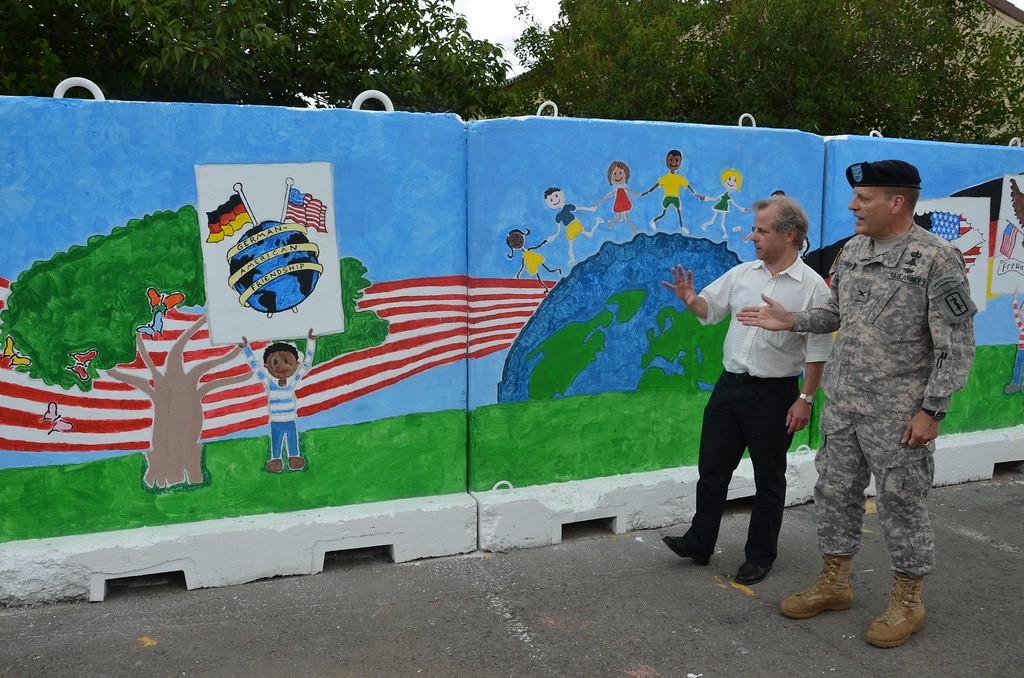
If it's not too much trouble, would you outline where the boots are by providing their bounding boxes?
[781,553,852,618]
[865,572,925,648]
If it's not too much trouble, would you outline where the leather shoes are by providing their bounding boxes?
[662,536,711,565]
[734,559,773,584]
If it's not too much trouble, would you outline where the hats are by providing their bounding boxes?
[846,160,922,189]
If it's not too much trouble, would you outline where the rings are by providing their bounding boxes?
[922,441,930,446]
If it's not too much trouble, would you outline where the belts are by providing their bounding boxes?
[723,369,798,385]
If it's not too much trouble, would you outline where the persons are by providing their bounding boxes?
[662,159,977,648]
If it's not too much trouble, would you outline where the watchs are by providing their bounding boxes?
[800,392,814,404]
[922,409,947,421]
